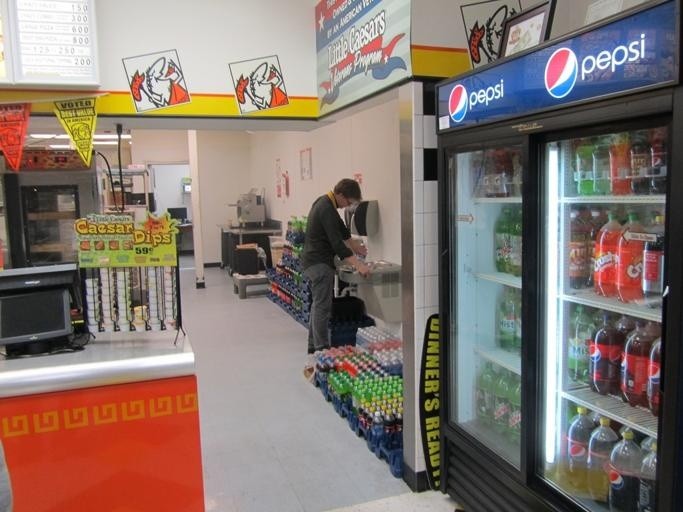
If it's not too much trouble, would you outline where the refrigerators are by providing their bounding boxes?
[432,0,681,508]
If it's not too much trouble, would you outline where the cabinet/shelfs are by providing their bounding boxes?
[220,217,282,275]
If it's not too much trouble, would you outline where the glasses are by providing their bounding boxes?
[347,199,352,206]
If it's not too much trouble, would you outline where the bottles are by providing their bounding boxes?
[609,131,631,195]
[615,315,634,334]
[567,406,597,495]
[286,215,308,249]
[576,137,597,195]
[619,320,657,410]
[472,147,524,198]
[588,317,603,393]
[647,333,663,418]
[587,417,622,504]
[640,435,657,453]
[584,306,595,318]
[593,313,626,395]
[567,305,597,384]
[509,207,524,278]
[629,130,651,195]
[651,127,669,194]
[305,326,406,480]
[591,411,602,427]
[593,134,612,195]
[598,209,613,228]
[514,290,522,350]
[642,216,666,309]
[283,244,304,275]
[508,375,521,446]
[594,213,623,298]
[645,211,660,226]
[615,213,644,304]
[476,362,497,427]
[638,443,658,512]
[608,431,641,512]
[266,265,311,323]
[492,206,517,274]
[569,207,600,289]
[498,286,517,352]
[626,320,643,345]
[491,369,514,435]
[571,138,579,196]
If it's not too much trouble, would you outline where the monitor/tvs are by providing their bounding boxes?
[167,207,187,219]
[1,262,87,332]
[0,288,73,354]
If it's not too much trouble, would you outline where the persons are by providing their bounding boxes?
[302,179,370,354]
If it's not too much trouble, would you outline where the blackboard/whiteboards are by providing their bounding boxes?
[146,162,195,253]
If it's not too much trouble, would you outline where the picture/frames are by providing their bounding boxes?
[496,0,557,58]
